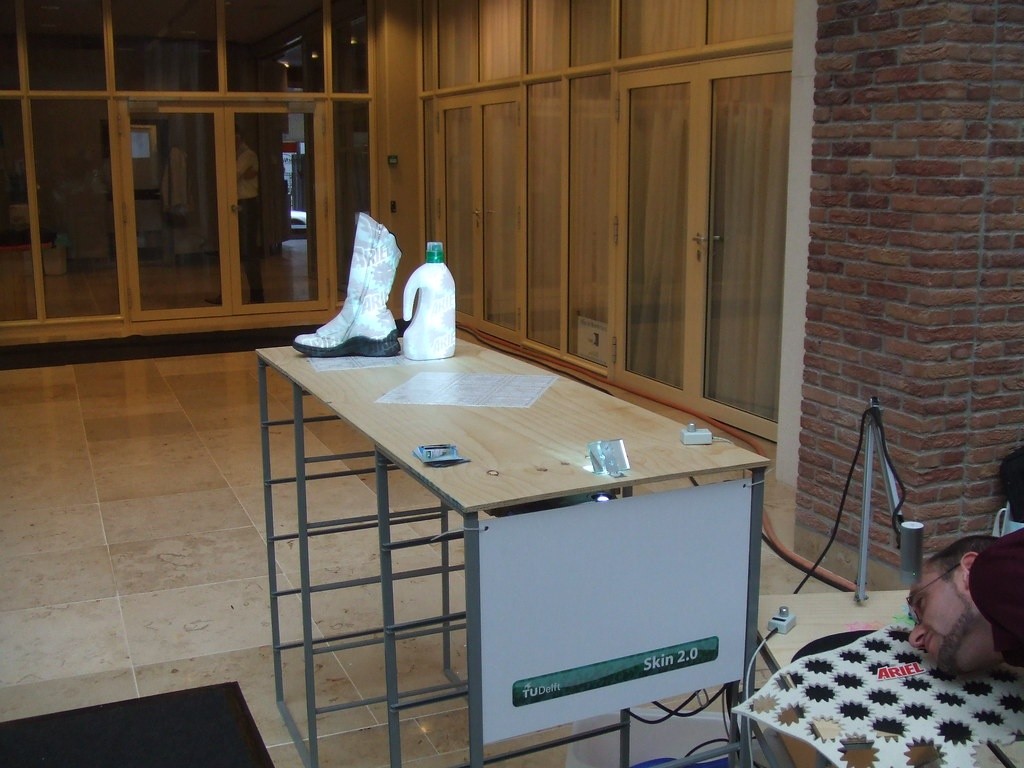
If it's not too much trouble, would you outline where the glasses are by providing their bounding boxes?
[906,563,961,626]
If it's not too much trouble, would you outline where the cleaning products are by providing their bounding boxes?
[401,239,458,362]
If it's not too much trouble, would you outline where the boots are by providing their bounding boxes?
[292,212,402,358]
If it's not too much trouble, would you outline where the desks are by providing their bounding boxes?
[262,337,771,768]
[757,590,1024,768]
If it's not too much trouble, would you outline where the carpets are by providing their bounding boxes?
[0,681,274,768]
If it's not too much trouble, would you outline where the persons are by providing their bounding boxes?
[907,527,1024,678]
[204,122,266,306]
